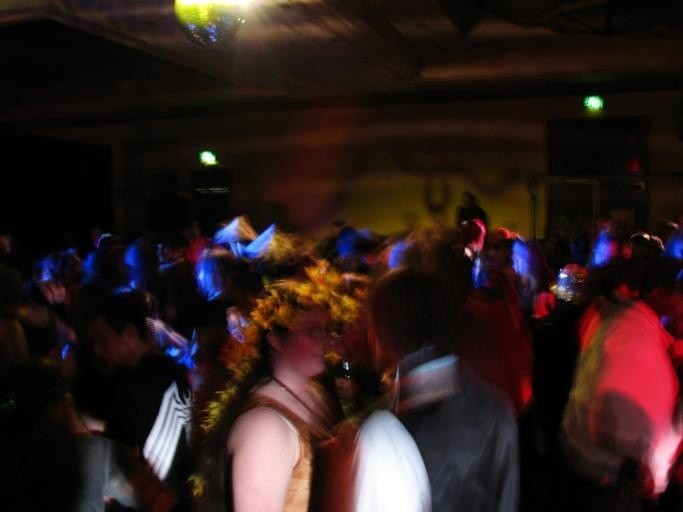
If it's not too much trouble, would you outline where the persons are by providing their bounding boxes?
[0,154,683,512]
